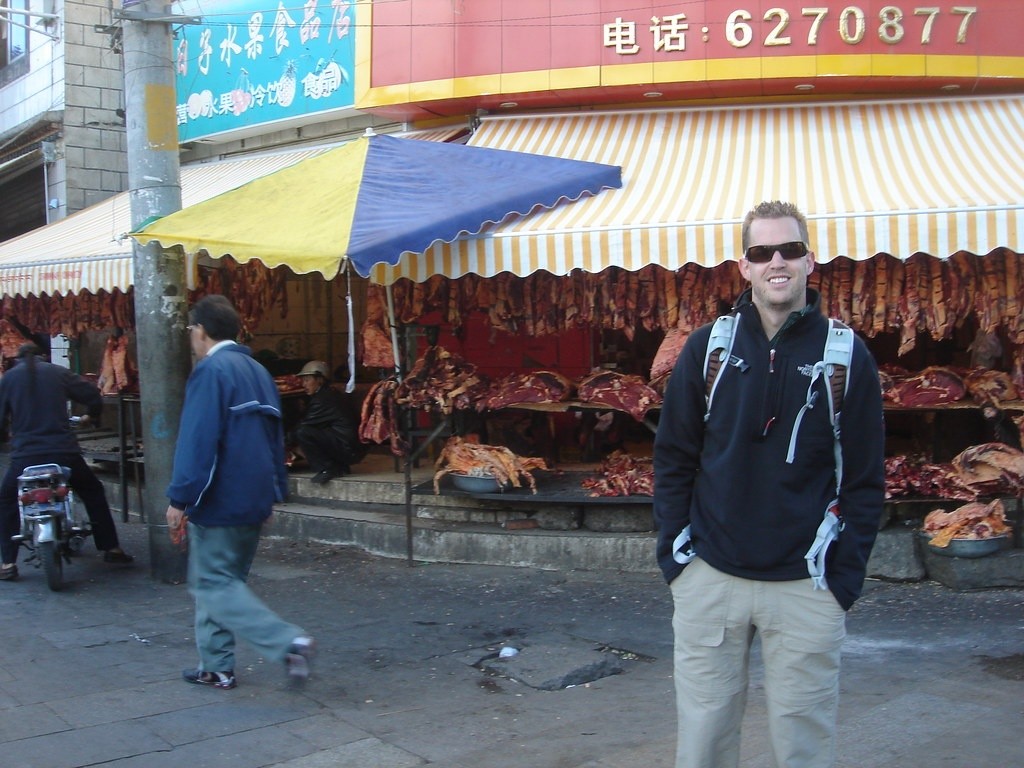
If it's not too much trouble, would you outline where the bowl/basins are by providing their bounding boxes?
[928,534,1009,559]
[450,472,518,493]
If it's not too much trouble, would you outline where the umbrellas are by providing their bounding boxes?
[109,126,623,382]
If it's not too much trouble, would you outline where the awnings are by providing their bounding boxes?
[0,124,465,300]
[369,96,1024,285]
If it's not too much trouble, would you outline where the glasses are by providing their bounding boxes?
[185,323,200,336]
[742,241,809,264]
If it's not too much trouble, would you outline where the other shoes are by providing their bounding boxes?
[102,550,133,566]
[0,564,19,581]
[311,468,339,482]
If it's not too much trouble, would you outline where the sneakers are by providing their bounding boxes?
[181,667,235,689]
[276,636,318,693]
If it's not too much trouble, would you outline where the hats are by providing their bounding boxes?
[296,361,332,382]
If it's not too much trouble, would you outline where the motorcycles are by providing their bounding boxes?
[11,415,88,592]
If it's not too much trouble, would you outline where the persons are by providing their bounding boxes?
[653,203,881,768]
[285,360,366,484]
[166,295,314,689]
[0,342,133,580]
